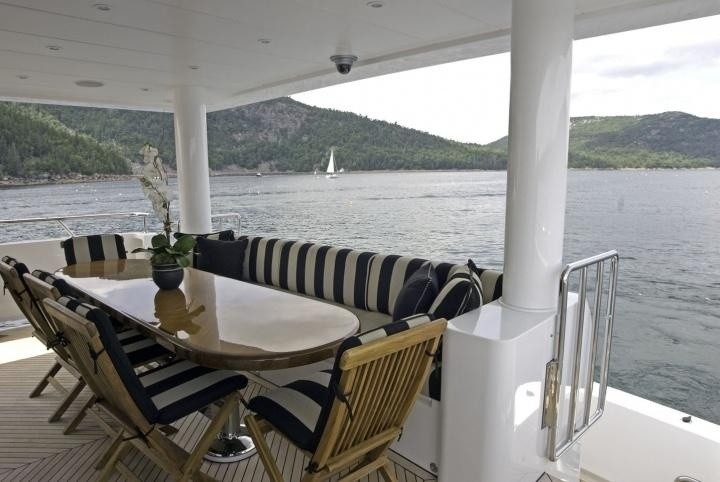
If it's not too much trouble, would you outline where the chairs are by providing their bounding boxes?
[244,313,447,482]
[23,268,176,423]
[59,234,127,264]
[1,257,44,343]
[42,294,249,481]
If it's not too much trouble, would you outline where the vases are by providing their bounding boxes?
[150,262,184,290]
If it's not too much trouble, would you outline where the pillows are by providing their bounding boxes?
[393,264,437,321]
[193,234,248,281]
[174,230,234,251]
[427,259,483,363]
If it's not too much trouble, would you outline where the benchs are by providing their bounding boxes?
[188,234,504,478]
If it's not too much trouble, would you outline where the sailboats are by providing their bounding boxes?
[325,149,340,179]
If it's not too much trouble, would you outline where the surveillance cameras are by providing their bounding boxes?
[330,55,358,75]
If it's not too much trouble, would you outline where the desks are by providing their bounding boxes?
[55,258,361,463]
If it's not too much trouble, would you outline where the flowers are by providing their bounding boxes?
[125,141,203,268]
